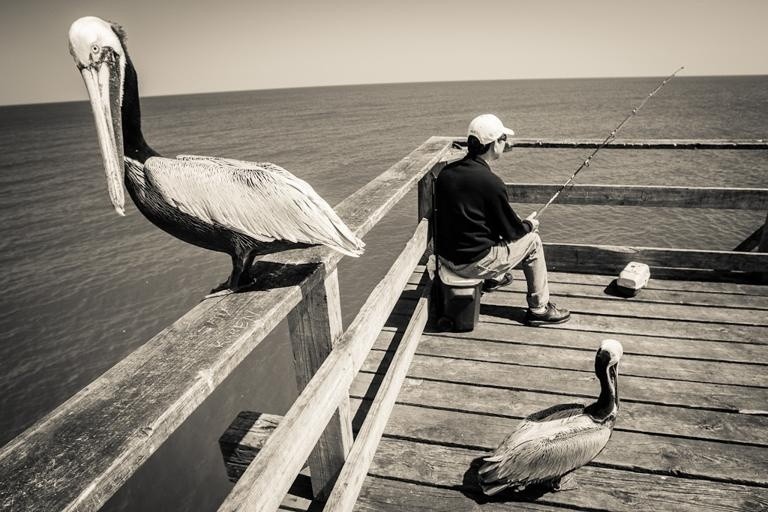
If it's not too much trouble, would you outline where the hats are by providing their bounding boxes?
[466,113,515,145]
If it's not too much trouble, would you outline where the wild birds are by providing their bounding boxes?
[67,14,368,303]
[473,336,624,498]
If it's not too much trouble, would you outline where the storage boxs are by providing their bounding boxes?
[614,260,653,297]
[438,260,488,331]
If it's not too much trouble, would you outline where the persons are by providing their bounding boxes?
[430,113,571,327]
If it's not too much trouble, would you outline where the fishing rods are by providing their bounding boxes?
[535,65,683,220]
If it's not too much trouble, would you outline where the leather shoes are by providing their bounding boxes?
[525,302,571,327]
[483,273,513,292]
